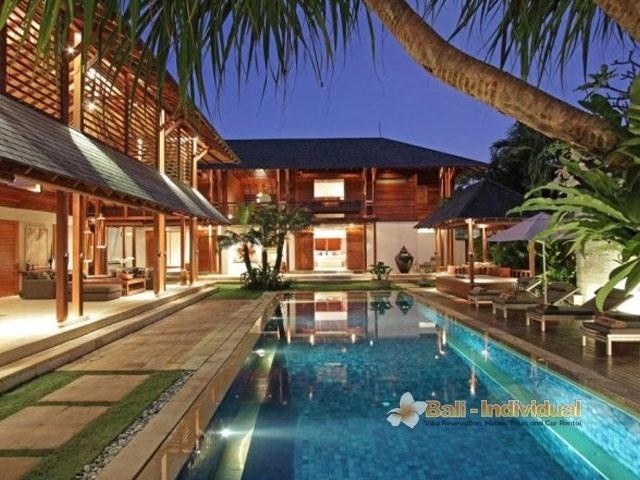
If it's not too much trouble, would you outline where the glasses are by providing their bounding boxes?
[96,200,106,220]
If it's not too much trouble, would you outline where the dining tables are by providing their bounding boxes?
[368,261,393,285]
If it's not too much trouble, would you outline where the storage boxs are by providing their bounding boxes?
[66,283,122,301]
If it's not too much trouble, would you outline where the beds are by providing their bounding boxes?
[487,210,571,305]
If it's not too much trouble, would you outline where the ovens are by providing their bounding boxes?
[435,272,519,300]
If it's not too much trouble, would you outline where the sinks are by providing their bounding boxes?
[21,269,72,299]
[117,267,153,290]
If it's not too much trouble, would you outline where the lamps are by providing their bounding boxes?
[579,315,640,355]
[492,282,580,319]
[467,278,543,309]
[525,286,634,332]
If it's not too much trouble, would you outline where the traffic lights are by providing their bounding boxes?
[447,261,512,277]
[34,271,57,280]
[123,267,152,280]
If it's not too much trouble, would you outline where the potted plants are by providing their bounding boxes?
[24,225,49,265]
